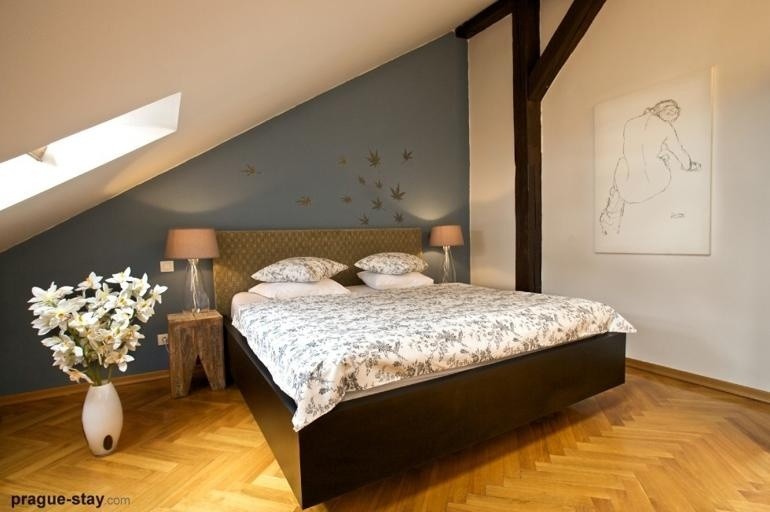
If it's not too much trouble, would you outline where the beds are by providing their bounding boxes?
[210,223,638,511]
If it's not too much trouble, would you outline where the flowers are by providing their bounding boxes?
[28,262,171,383]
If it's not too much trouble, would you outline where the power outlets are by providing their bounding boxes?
[156,332,169,346]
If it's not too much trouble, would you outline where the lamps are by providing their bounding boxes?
[163,226,222,313]
[427,225,465,283]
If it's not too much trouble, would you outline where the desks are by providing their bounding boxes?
[165,308,228,398]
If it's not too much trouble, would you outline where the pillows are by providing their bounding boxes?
[353,251,431,276]
[248,278,352,300]
[354,270,437,291]
[248,255,351,283]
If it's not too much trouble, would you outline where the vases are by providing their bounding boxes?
[80,385,126,457]
